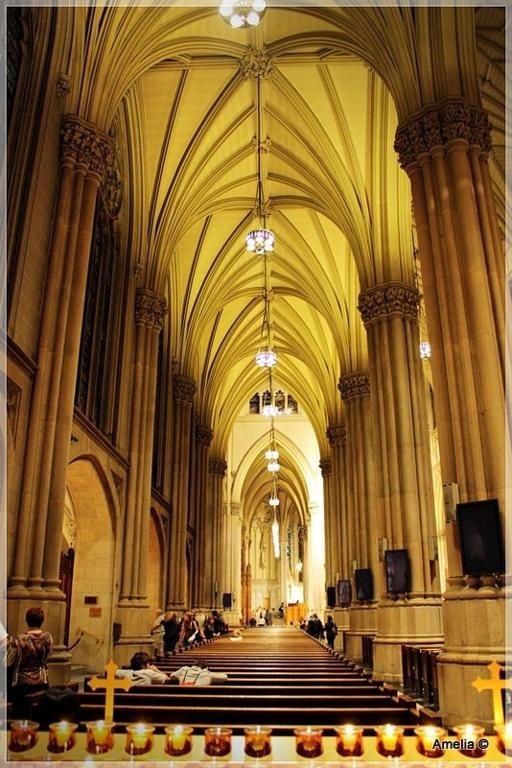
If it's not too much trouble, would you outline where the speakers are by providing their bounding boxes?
[327,587,335,606]
[385,548,410,593]
[223,593,232,607]
[355,568,373,600]
[338,580,351,604]
[456,498,505,576]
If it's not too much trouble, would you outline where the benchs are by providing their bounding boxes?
[5,621,445,734]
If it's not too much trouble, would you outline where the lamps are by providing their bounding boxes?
[217,0,281,419]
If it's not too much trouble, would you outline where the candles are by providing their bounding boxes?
[8,718,512,755]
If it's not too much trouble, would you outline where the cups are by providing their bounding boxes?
[11,717,511,759]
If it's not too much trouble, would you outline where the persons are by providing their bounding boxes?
[115,652,166,685]
[170,659,228,687]
[150,600,337,654]
[1,608,54,720]
